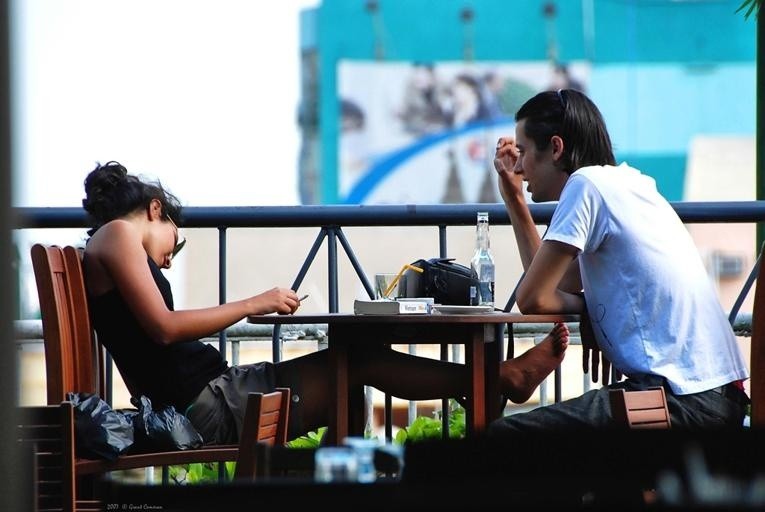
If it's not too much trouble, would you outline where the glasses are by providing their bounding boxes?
[167,214,186,259]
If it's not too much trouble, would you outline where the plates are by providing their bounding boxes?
[432,305,494,314]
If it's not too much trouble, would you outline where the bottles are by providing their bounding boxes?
[470,211,494,312]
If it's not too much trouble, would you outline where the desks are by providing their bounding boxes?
[248,306,583,448]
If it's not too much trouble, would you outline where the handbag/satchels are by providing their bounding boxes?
[398,258,482,306]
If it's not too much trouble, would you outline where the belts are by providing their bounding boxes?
[713,384,751,407]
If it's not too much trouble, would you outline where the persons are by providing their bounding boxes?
[395,62,486,135]
[340,100,364,134]
[548,66,584,93]
[83,161,570,449]
[490,89,749,483]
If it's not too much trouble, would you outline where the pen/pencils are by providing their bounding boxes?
[298,295,309,302]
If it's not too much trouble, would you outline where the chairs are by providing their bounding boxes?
[14,245,292,512]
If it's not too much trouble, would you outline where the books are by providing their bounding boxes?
[354,299,427,316]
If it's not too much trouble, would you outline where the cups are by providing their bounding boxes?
[375,274,408,301]
